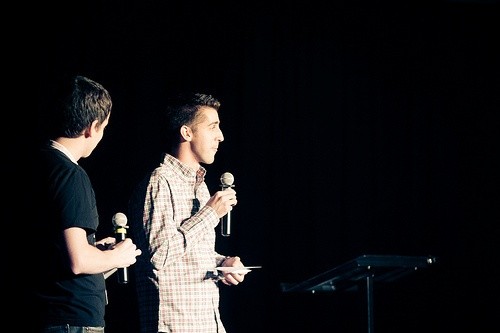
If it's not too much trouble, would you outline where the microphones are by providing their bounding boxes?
[220,172,234,237]
[112,213,129,283]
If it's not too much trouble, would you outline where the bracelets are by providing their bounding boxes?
[218,255,231,287]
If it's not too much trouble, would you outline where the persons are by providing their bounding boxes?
[0,78,142,333]
[142,93,249,333]
